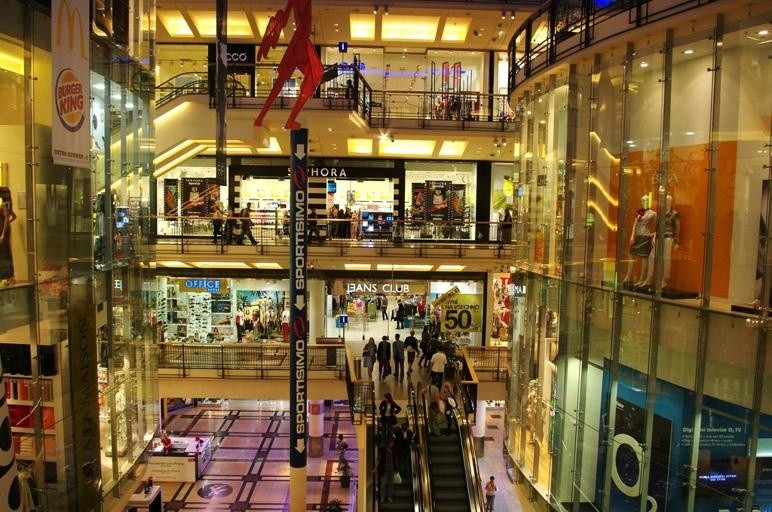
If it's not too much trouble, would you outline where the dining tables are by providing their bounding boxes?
[335,458,359,488]
[319,498,348,512]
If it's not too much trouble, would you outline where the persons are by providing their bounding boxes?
[236,309,244,343]
[395,300,404,329]
[434,97,472,120]
[161,435,173,455]
[362,325,457,505]
[0,185,17,286]
[211,202,259,246]
[283,205,351,243]
[622,195,657,287]
[214,327,220,339]
[98,204,116,264]
[499,208,513,244]
[345,79,353,99]
[484,476,498,512]
[336,434,350,470]
[380,295,389,320]
[638,194,680,289]
[281,308,290,342]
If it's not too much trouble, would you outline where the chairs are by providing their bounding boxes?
[380,496,386,504]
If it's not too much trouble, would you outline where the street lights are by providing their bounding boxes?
[213,214,222,226]
[364,356,373,367]
[384,361,391,376]
[393,471,402,485]
[430,402,437,410]
[434,412,446,424]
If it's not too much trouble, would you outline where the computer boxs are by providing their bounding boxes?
[391,136,394,142]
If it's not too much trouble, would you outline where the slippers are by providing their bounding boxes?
[239,209,250,225]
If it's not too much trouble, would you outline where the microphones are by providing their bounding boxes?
[403,239,476,258]
[157,234,223,254]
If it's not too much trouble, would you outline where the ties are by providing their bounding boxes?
[360,210,394,237]
[328,183,336,193]
[212,301,231,312]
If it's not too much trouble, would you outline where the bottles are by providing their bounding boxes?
[384,436,394,446]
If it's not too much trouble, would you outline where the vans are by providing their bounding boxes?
[4,277,13,286]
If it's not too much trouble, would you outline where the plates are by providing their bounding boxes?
[1,309,74,512]
[167,285,233,339]
[143,276,167,342]
